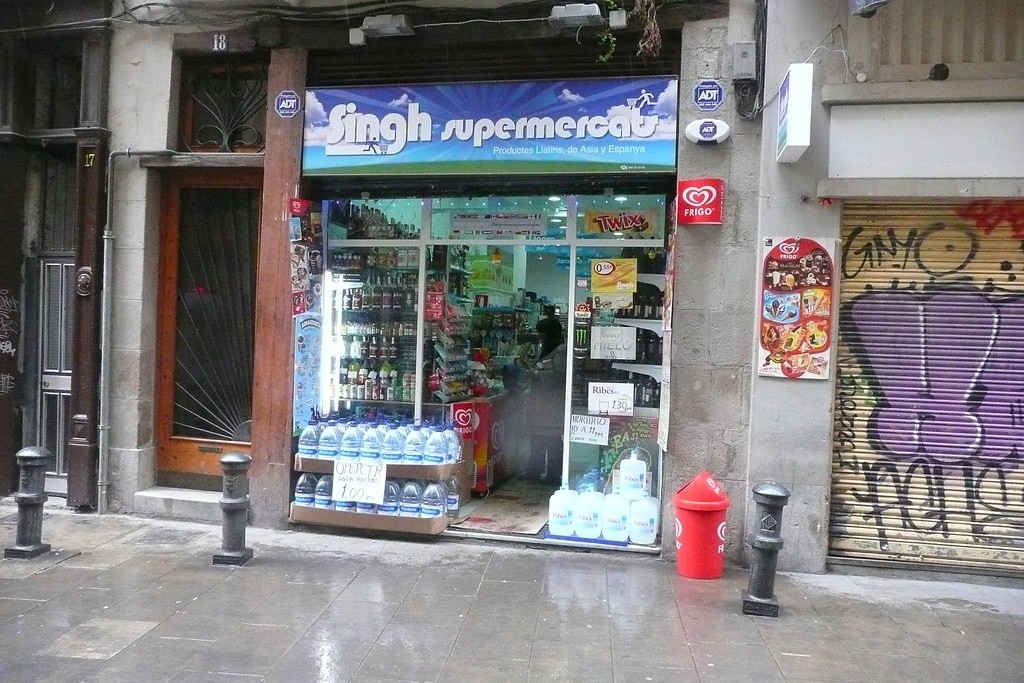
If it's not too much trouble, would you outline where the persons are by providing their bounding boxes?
[517,317,577,484]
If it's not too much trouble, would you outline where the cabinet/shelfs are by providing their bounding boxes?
[327,216,532,502]
[609,272,665,418]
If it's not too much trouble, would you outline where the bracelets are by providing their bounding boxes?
[534,370,538,376]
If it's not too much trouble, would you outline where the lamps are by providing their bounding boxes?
[546,3,603,28]
[360,16,415,38]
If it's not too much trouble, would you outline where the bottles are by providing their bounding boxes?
[615,287,664,319]
[294,473,463,519]
[622,236,666,274]
[331,313,417,402]
[328,246,419,311]
[347,204,419,239]
[548,450,658,544]
[298,414,461,464]
[636,329,663,364]
[625,371,661,407]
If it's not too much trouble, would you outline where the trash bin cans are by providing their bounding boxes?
[674,472,730,580]
